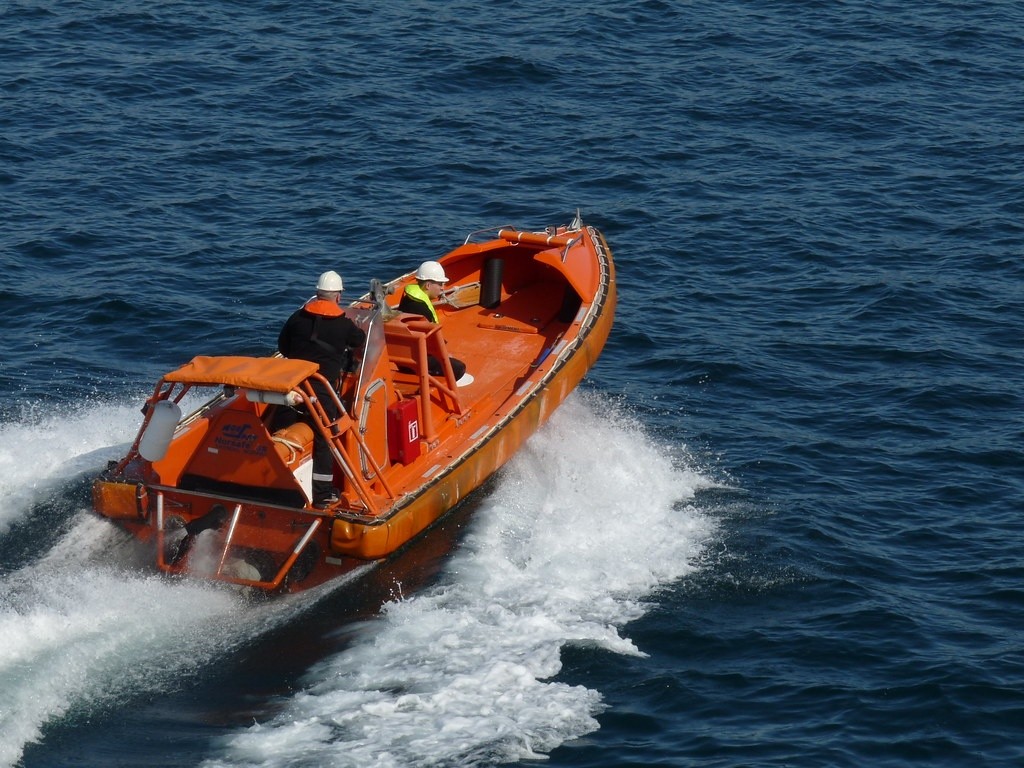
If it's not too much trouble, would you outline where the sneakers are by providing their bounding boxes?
[314,488,340,509]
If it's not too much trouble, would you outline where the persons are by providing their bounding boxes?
[398,260,466,382]
[278,271,367,505]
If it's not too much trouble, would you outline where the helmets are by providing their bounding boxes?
[316,271,346,292]
[414,261,449,283]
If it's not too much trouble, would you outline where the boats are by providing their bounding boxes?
[87,207,619,593]
